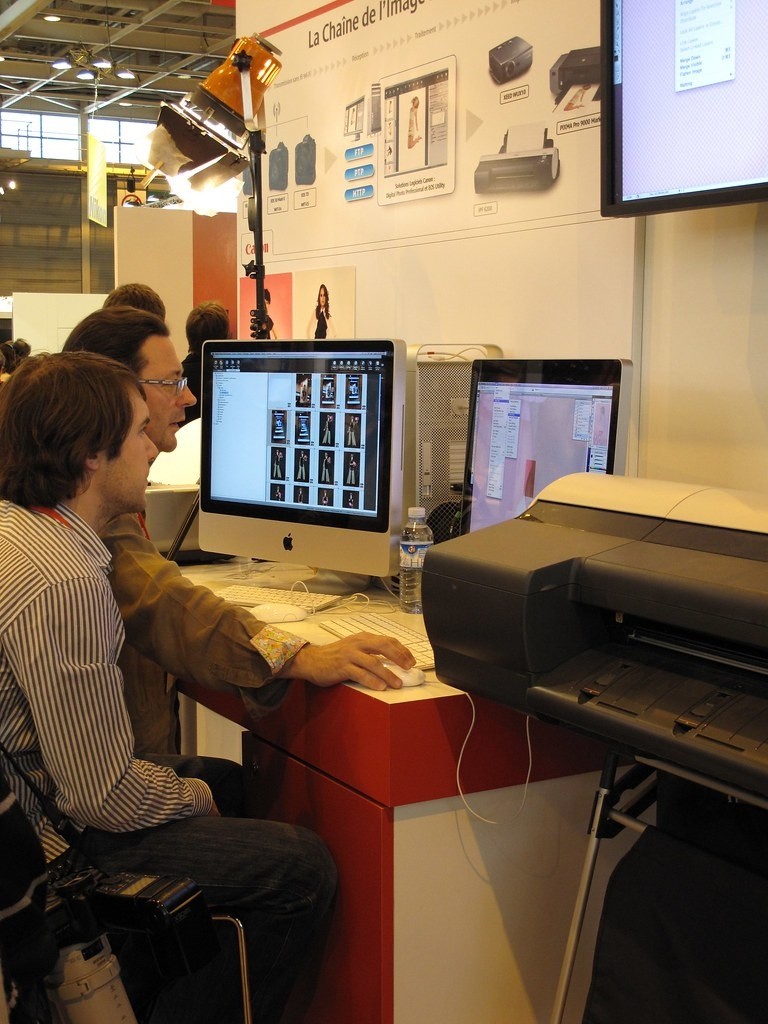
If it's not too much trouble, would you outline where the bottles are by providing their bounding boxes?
[399,505,434,615]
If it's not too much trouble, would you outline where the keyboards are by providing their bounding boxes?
[214,584,343,614]
[317,612,436,671]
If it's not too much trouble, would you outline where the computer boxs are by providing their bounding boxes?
[368,343,504,590]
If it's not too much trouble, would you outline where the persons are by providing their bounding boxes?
[1,285,416,1023]
[180,301,228,427]
[306,284,336,339]
[408,97,422,150]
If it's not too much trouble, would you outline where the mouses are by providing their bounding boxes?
[250,603,308,623]
[368,662,426,687]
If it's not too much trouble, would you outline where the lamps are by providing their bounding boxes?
[145,31,283,341]
[126,166,136,193]
[52,59,136,82]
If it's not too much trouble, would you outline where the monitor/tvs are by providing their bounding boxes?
[200,339,409,598]
[459,357,633,537]
[600,0,768,218]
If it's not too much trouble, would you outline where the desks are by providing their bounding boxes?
[166,564,657,1024]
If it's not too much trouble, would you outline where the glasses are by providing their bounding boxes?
[135,377,188,396]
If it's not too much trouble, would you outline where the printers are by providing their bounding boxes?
[420,470,768,801]
[549,46,600,103]
[473,129,561,194]
